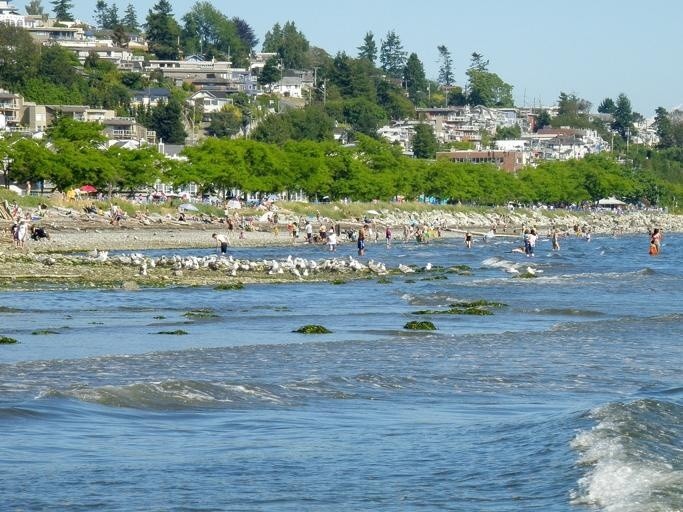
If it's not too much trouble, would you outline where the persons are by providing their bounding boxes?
[404,212,447,242]
[464,231,473,248]
[137,193,167,204]
[267,212,299,243]
[176,213,223,225]
[25,180,32,195]
[582,203,635,213]
[376,227,392,245]
[38,201,42,211]
[7,199,29,249]
[225,216,247,239]
[358,224,369,256]
[304,221,312,243]
[316,209,321,220]
[490,222,526,233]
[313,223,337,250]
[524,219,564,257]
[650,227,660,255]
[574,223,593,243]
[67,187,104,216]
[212,233,232,256]
